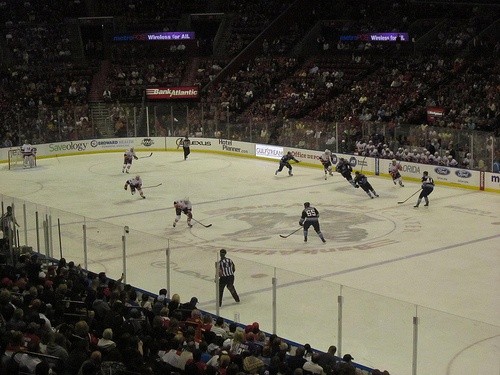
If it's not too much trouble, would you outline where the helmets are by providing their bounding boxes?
[304,202,310,207]
[383,144,450,158]
[325,149,330,153]
[392,159,396,164]
[184,195,189,201]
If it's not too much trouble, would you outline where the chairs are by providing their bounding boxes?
[22,299,89,375]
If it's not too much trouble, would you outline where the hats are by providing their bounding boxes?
[191,297,199,303]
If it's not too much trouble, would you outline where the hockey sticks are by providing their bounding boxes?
[398,188,422,204]
[279,226,303,238]
[141,183,162,189]
[182,211,212,228]
[134,152,153,160]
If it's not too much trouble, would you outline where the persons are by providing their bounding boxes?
[124,176,146,198]
[388,159,405,187]
[335,157,358,188]
[299,202,326,243]
[21,140,36,168]
[319,149,334,182]
[183,136,191,159]
[173,197,192,227]
[214,249,240,307]
[0,206,20,243]
[354,171,379,199]
[0,239,390,375]
[0,0,500,173]
[122,147,138,174]
[275,151,299,176]
[414,171,435,207]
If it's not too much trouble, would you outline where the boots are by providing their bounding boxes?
[321,237,326,243]
[304,236,307,241]
[372,191,379,197]
[368,193,374,199]
[424,201,429,206]
[413,202,419,207]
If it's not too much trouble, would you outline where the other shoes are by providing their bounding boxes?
[324,175,327,180]
[399,181,404,187]
[394,182,396,185]
[288,170,293,176]
[275,169,281,175]
[329,173,333,176]
[141,196,145,198]
[187,222,192,227]
[173,222,176,227]
